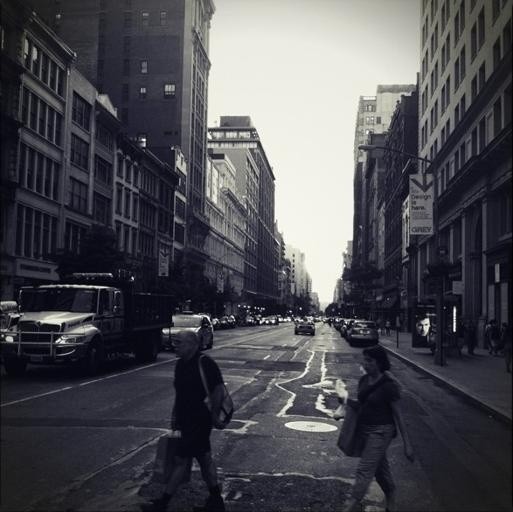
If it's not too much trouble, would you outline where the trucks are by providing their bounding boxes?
[0,274,174,377]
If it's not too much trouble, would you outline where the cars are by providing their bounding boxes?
[340,319,378,346]
[211,315,236,330]
[295,319,315,335]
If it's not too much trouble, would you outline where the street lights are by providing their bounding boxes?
[357,144,446,367]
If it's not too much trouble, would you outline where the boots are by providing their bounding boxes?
[192,484,228,512]
[140,492,171,512]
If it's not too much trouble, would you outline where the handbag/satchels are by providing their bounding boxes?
[152,434,191,482]
[204,384,233,430]
[337,403,369,458]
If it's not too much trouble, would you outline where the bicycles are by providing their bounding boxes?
[456,318,512,373]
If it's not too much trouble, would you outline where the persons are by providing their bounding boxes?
[375,314,386,336]
[498,322,513,372]
[139,328,227,512]
[414,313,432,347]
[463,318,478,354]
[487,320,498,357]
[384,315,392,337]
[332,342,419,512]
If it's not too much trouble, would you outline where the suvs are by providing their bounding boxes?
[161,314,214,352]
